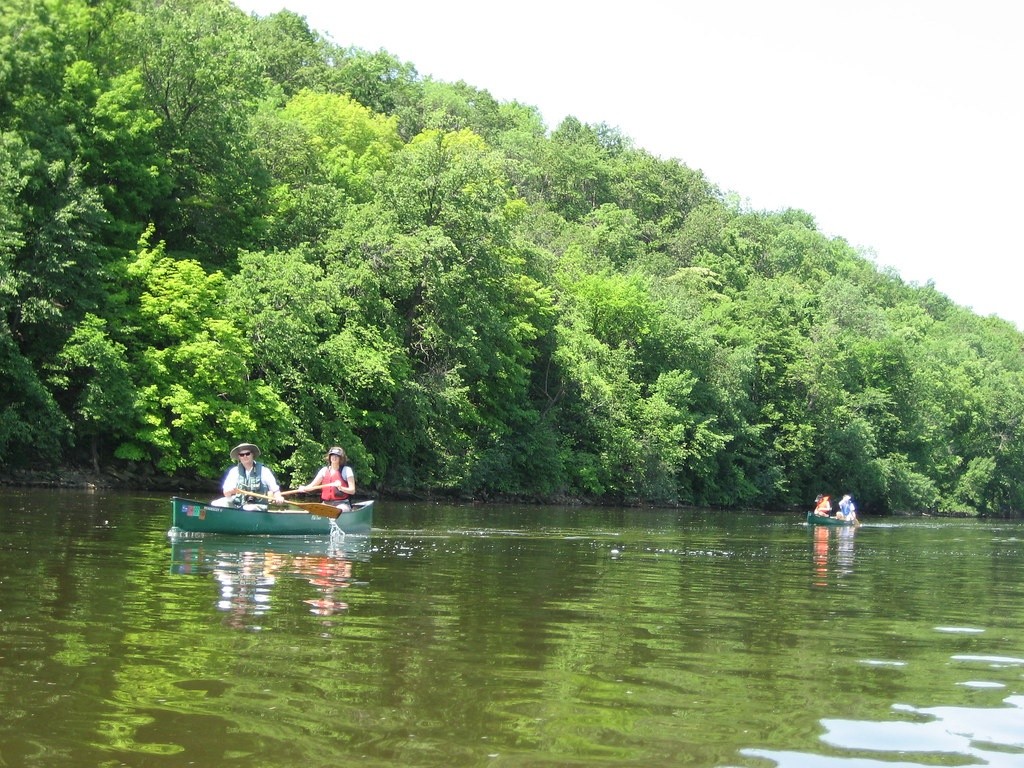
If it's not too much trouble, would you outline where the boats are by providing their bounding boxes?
[168,494,375,536]
[806,510,860,526]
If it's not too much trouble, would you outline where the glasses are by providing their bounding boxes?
[237,452,250,457]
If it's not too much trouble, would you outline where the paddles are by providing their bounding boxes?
[281,482,335,496]
[236,488,342,520]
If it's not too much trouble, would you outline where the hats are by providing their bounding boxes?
[230,442,261,462]
[329,447,344,456]
[844,496,851,500]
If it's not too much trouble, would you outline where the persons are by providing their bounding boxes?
[837,494,856,521]
[300,447,356,512]
[211,443,284,512]
[814,494,832,518]
